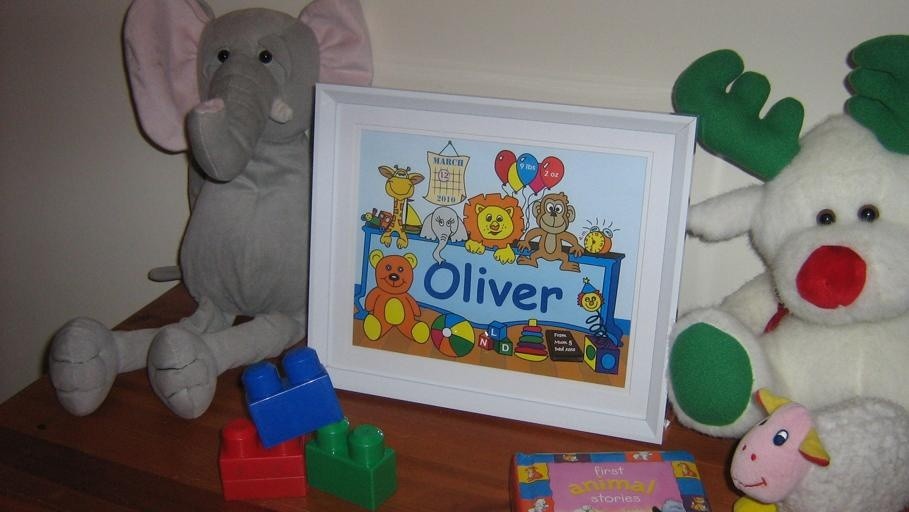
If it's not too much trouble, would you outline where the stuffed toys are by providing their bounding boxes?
[45,0,373,418]
[666,35,909,438]
[723,388,908,511]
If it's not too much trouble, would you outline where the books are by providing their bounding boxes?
[507,447,713,510]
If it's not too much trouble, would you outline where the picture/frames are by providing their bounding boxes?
[303,83,697,446]
